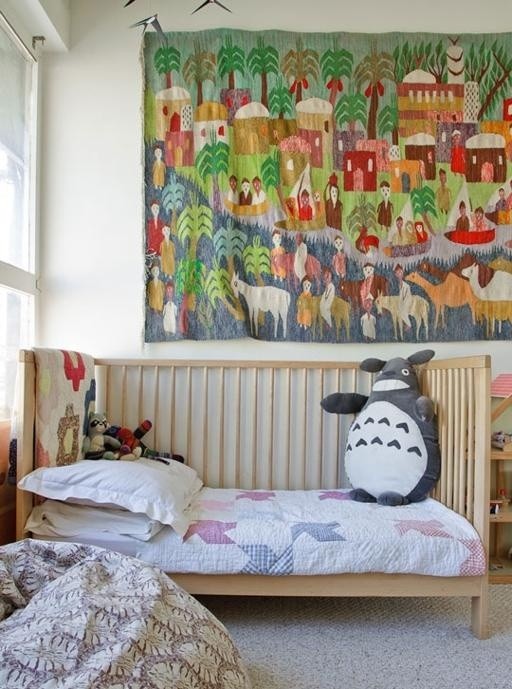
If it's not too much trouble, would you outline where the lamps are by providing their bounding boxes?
[490,374,512,400]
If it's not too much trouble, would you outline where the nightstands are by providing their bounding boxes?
[466,395,511,584]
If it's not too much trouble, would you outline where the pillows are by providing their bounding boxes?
[17,458,204,542]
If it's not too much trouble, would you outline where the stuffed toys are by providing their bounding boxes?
[106,419,184,461]
[320,347,442,506]
[82,412,121,461]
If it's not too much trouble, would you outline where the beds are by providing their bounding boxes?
[14,349,490,640]
